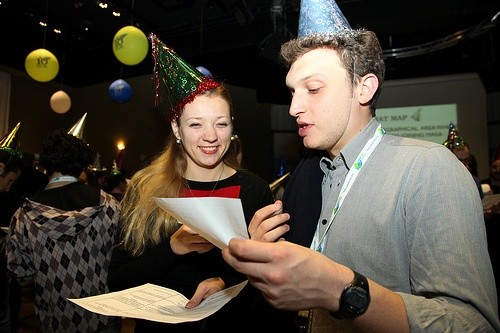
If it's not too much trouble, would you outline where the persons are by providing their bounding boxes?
[107,33,274,333]
[443,123,500,237]
[0,112,285,333]
[221,0,500,333]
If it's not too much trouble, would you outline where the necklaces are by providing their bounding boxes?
[183,163,225,198]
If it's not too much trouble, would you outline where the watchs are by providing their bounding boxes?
[328,269,371,324]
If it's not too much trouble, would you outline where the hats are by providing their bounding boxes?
[298,0,352,39]
[0,121,24,160]
[442,121,463,151]
[110,160,121,176]
[148,33,222,122]
[90,152,104,173]
[66,112,88,141]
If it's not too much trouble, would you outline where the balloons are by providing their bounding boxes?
[113,26,149,65]
[195,66,212,79]
[25,49,60,83]
[109,79,132,100]
[51,91,72,113]
